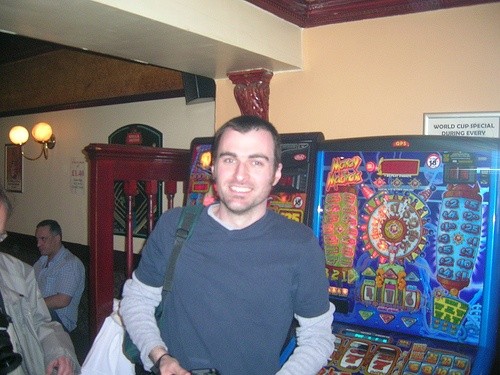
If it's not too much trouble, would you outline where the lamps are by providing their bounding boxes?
[9,122,56,160]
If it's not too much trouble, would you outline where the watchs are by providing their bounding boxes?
[150,353,174,375]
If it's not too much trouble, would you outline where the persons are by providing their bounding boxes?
[0,190,86,375]
[118,115,337,375]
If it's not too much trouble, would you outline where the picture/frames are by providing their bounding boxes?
[4,143,24,193]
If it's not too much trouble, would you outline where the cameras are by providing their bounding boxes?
[0,305,23,375]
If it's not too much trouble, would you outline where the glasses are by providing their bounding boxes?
[0,233,7,242]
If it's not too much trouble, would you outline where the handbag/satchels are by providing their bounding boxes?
[123,205,203,364]
